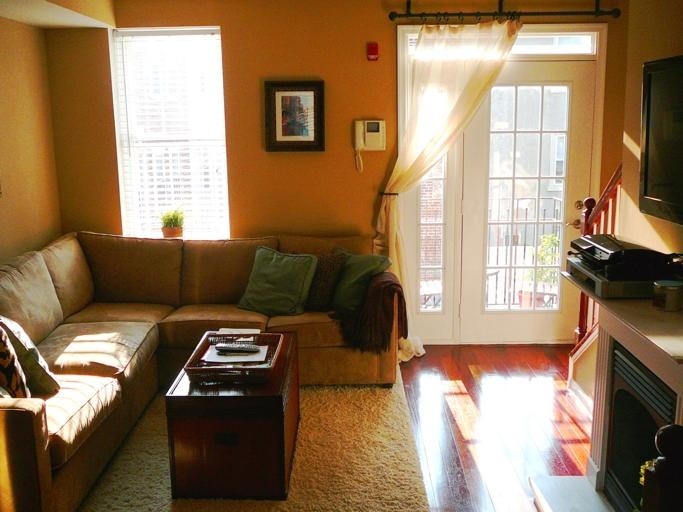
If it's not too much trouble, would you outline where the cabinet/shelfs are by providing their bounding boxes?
[559,271,683,512]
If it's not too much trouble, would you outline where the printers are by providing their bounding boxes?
[571,234,673,280]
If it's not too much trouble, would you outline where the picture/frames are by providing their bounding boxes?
[265,79,325,150]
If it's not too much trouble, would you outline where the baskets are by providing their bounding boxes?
[182,329,287,387]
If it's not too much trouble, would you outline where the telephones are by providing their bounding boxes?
[355,120,386,151]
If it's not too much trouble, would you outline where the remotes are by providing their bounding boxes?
[216,343,259,351]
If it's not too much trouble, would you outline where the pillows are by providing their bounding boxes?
[238,246,316,316]
[286,247,343,310]
[329,246,392,312]
[1,317,59,400]
[1,325,32,400]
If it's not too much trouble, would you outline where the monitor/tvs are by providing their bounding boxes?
[639,56,683,224]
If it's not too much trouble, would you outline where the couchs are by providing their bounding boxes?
[157,234,398,388]
[0,229,183,511]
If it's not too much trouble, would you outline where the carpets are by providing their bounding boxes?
[70,385,429,510]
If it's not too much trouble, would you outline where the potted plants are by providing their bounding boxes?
[162,212,185,238]
